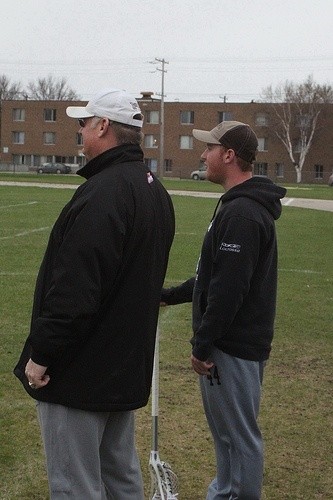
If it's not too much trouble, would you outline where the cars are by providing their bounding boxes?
[36,163,71,174]
[190,165,210,180]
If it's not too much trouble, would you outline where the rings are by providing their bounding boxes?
[28,382,36,389]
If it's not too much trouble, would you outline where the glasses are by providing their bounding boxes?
[77,118,111,127]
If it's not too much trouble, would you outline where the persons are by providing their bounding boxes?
[158,121,288,499]
[12,87,179,500]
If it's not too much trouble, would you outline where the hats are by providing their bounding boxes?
[65,89,144,128]
[192,121,258,163]
